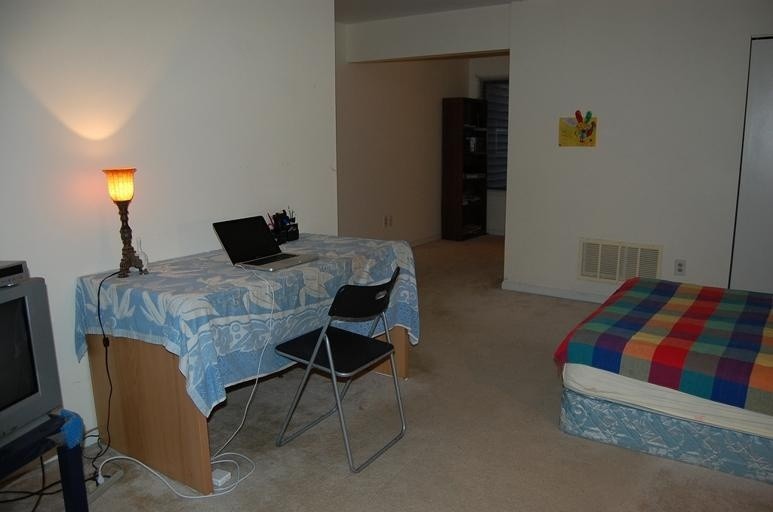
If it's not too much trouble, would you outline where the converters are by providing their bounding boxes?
[211,468,232,486]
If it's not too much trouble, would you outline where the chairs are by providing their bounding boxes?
[273,264,407,474]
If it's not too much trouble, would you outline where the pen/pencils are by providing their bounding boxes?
[269,207,297,231]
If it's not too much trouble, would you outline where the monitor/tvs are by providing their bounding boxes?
[0,276,63,451]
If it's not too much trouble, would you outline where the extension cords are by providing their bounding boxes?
[85,462,124,504]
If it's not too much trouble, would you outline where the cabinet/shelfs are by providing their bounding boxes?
[441,97,489,242]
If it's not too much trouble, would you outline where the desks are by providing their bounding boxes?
[1,415,94,510]
[79,232,415,497]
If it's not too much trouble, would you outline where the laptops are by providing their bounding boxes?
[212,214,320,273]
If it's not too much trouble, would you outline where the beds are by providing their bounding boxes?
[556,274,773,487]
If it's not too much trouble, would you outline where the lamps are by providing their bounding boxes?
[103,166,148,280]
